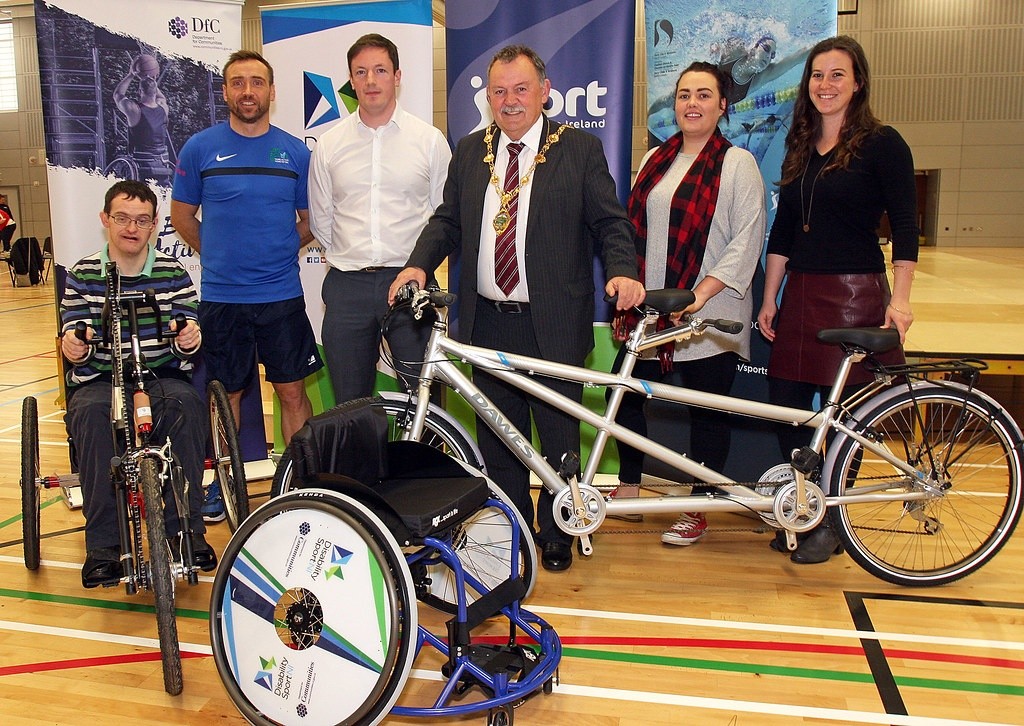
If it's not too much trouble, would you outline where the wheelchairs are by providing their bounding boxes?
[208,397,562,726]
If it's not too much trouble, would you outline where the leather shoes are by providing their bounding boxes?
[190,532,212,564]
[541,542,572,572]
[87,563,124,582]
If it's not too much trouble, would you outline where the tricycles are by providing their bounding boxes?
[19,260,249,695]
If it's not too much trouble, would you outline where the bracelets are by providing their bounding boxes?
[889,304,912,315]
[891,265,915,280]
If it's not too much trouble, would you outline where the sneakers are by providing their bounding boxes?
[602,490,644,521]
[660,509,708,545]
[200,476,238,522]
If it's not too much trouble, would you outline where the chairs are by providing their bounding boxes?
[41,236,53,282]
[0,236,45,288]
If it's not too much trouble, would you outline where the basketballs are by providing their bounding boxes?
[136,54,160,79]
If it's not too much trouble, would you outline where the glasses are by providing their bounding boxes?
[108,213,154,230]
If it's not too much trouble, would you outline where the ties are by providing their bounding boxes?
[494,142,526,298]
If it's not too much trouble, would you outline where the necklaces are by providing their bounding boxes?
[484,125,574,235]
[801,149,836,232]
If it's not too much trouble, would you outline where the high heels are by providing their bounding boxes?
[791,505,845,564]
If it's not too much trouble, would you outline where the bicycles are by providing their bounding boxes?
[271,279,1024,588]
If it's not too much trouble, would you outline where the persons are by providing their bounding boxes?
[0,196,16,252]
[305,33,455,412]
[57,182,218,585]
[757,39,919,564]
[114,65,170,167]
[388,45,646,571]
[602,64,767,544]
[171,51,326,524]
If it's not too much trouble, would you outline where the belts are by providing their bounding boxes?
[478,294,531,315]
